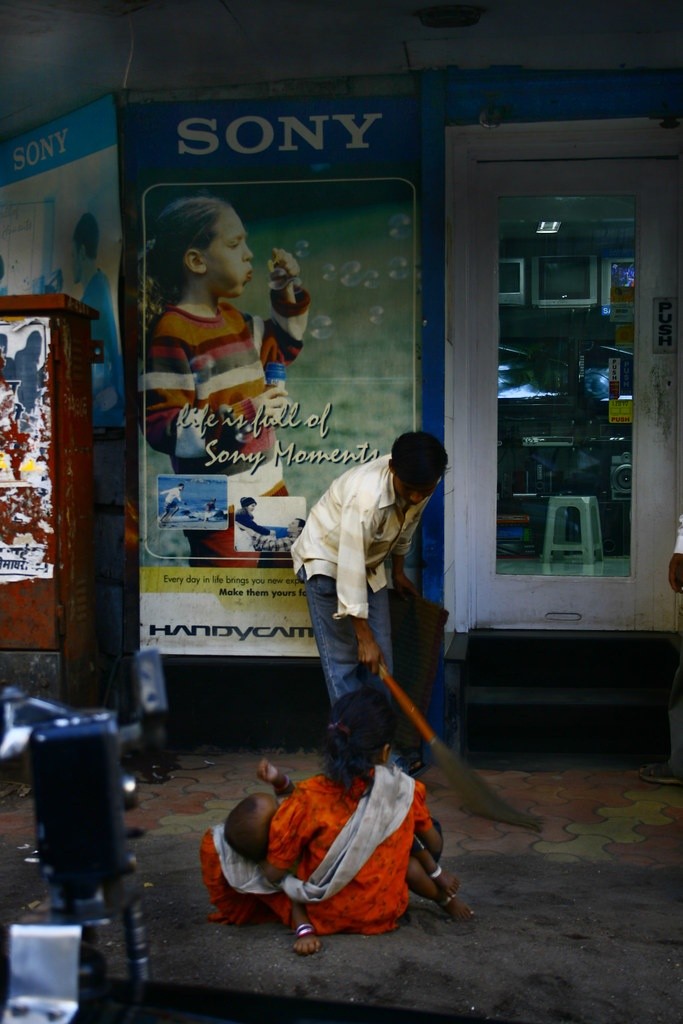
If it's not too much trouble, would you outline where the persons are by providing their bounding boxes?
[225,689,475,955]
[139,196,312,568]
[234,497,306,552]
[638,513,682,784]
[159,483,188,523]
[289,431,448,779]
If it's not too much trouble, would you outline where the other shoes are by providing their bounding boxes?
[638,763,683,785]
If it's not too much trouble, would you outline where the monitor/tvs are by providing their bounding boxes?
[497,337,609,416]
[531,256,598,307]
[498,258,525,305]
[601,257,635,307]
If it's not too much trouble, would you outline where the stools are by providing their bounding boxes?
[542,495,603,564]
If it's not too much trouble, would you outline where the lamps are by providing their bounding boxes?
[413,6,486,28]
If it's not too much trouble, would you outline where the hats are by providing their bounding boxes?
[240,497,257,507]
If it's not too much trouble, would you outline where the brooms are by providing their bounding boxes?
[377,659,550,833]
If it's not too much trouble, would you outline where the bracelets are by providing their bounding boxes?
[275,773,289,792]
[296,923,315,939]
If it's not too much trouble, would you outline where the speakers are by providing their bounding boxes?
[598,501,624,556]
[609,452,632,500]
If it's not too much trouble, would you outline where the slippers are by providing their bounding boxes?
[394,756,434,778]
